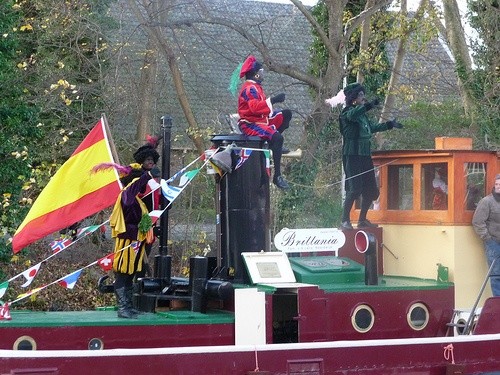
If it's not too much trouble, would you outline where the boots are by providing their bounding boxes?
[115,287,140,319]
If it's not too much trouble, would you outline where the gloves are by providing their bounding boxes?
[369,98,383,109]
[271,93,285,105]
[390,118,404,130]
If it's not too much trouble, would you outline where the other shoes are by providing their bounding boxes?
[357,219,378,228]
[342,220,354,230]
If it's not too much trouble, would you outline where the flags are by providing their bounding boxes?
[0,149,271,320]
[12,118,122,253]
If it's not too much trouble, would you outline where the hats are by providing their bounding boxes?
[240,55,257,78]
[133,143,160,164]
[120,169,144,187]
[343,83,364,104]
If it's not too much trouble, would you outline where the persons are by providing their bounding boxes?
[108,143,162,318]
[238,55,292,189]
[338,83,403,230]
[470,174,500,296]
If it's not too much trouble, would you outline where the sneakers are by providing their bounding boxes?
[273,174,290,190]
[282,147,289,155]
